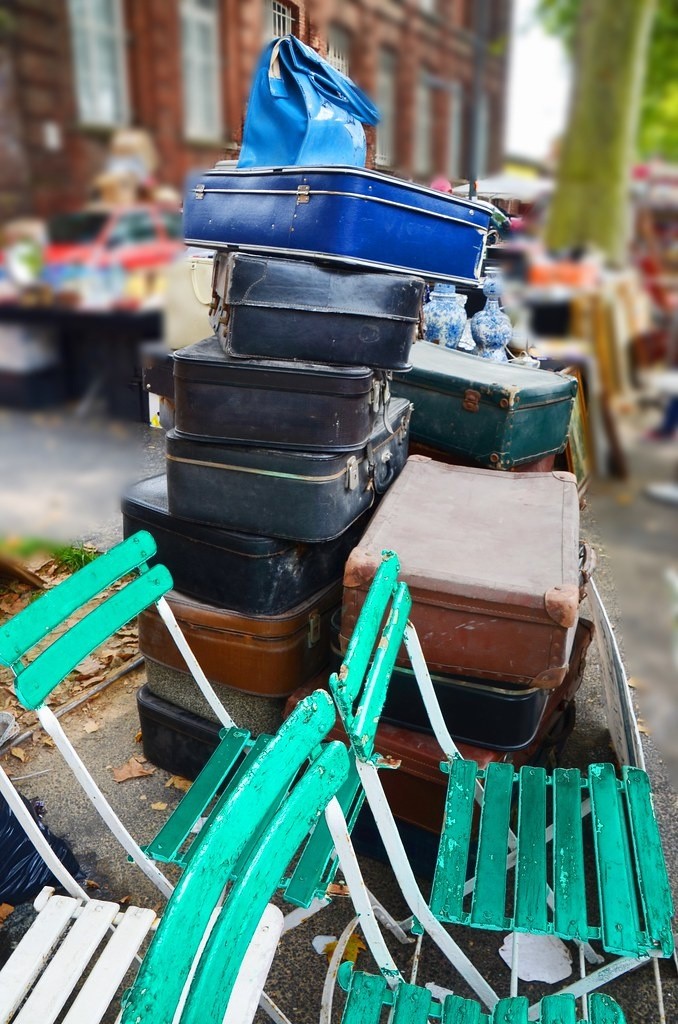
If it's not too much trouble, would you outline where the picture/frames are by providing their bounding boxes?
[556,365,596,499]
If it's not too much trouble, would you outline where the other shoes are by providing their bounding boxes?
[641,428,676,445]
[646,478,678,505]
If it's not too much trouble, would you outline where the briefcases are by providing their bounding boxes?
[117,164,509,800]
[388,338,580,472]
[275,454,596,898]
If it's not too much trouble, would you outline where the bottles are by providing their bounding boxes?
[470,266,513,364]
[420,281,469,350]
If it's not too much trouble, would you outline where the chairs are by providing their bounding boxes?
[2,530,677,1024]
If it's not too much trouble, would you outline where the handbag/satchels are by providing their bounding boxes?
[236,33,384,168]
